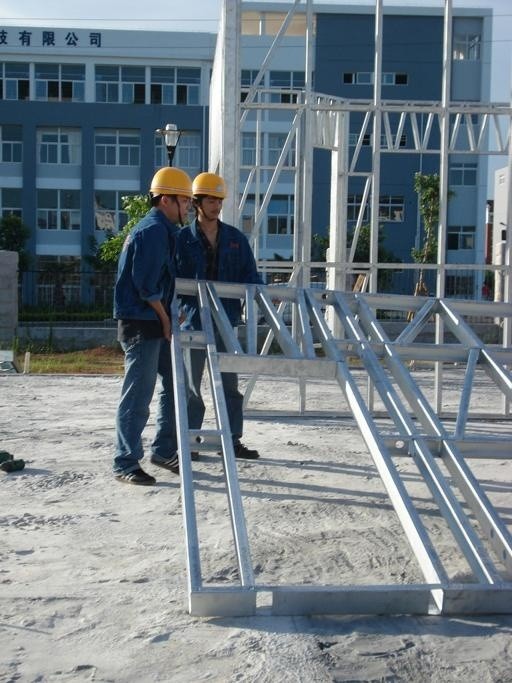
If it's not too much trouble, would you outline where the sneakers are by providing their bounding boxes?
[151,453,179,474]
[218,444,259,458]
[114,468,156,485]
[190,437,201,460]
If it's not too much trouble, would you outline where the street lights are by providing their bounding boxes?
[155,121,186,166]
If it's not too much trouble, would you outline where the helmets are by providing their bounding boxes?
[193,172,225,198]
[149,167,193,197]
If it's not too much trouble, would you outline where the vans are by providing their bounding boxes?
[259,279,327,324]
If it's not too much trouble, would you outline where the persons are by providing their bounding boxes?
[109,163,199,488]
[176,171,280,461]
